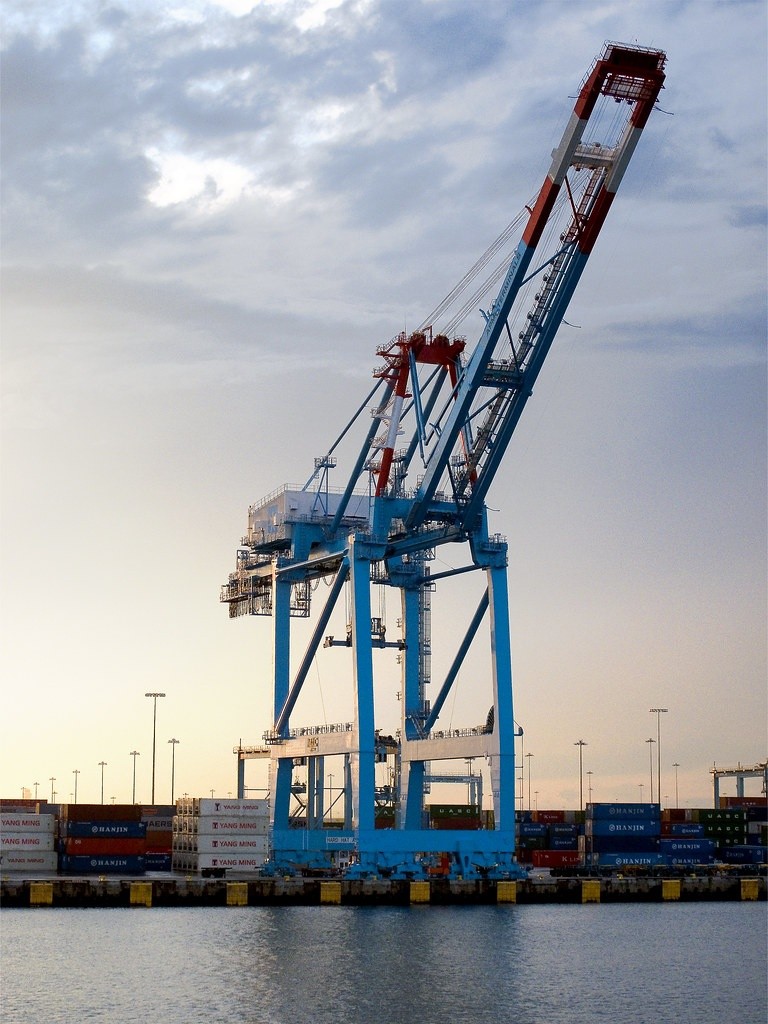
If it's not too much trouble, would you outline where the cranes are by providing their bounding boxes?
[220,35,671,881]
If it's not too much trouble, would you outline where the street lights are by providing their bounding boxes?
[525,752,534,810]
[387,766,393,807]
[647,706,669,804]
[466,761,472,805]
[672,762,684,811]
[534,791,540,810]
[573,740,588,812]
[145,692,167,804]
[587,770,595,804]
[665,795,669,808]
[73,769,80,804]
[33,782,40,798]
[326,774,336,820]
[168,738,181,805]
[130,750,140,804]
[645,738,657,802]
[49,777,57,803]
[21,787,27,799]
[210,789,215,798]
[638,783,644,803]
[98,762,108,803]
